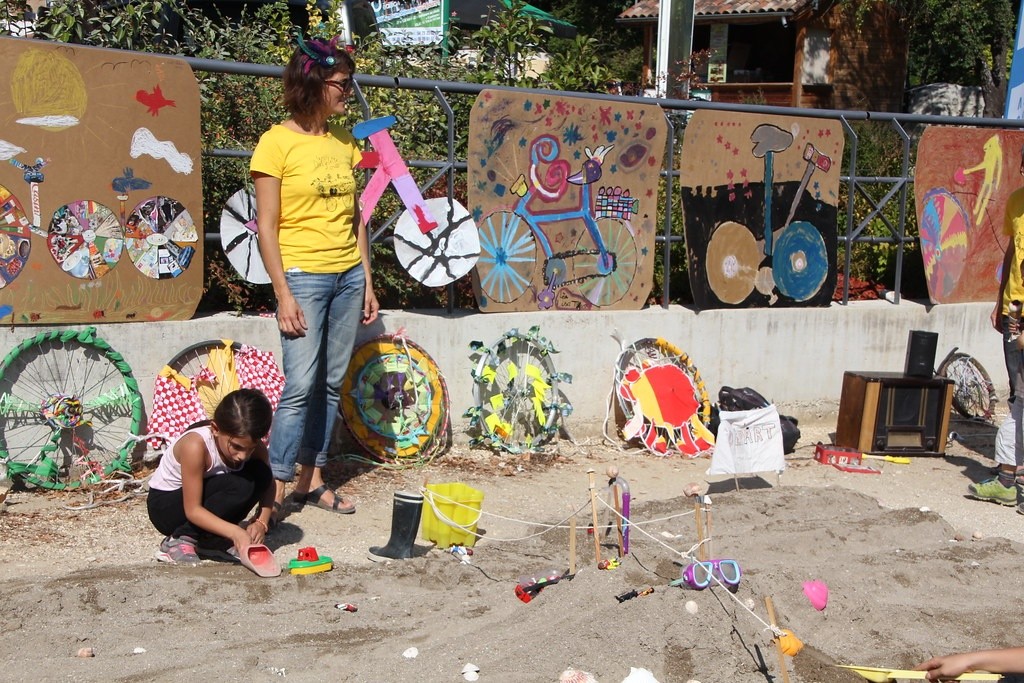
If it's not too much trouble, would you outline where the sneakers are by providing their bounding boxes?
[968,475,1017,505]
[1018,502,1024,514]
[155,536,202,567]
[195,541,240,561]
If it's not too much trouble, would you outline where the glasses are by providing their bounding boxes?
[670,559,741,590]
[324,78,354,92]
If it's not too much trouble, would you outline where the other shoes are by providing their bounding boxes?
[991,465,1024,485]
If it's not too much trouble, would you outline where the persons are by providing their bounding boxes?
[250,37,379,527]
[146,389,276,567]
[967,145,1024,515]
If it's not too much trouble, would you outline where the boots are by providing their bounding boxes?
[367,491,424,563]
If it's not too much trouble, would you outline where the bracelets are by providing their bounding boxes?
[251,518,268,533]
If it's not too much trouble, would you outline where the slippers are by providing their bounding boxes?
[293,485,355,513]
[251,500,285,534]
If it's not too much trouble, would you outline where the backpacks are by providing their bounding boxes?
[699,386,801,455]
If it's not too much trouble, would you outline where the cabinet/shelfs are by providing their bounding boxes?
[835,371,955,458]
